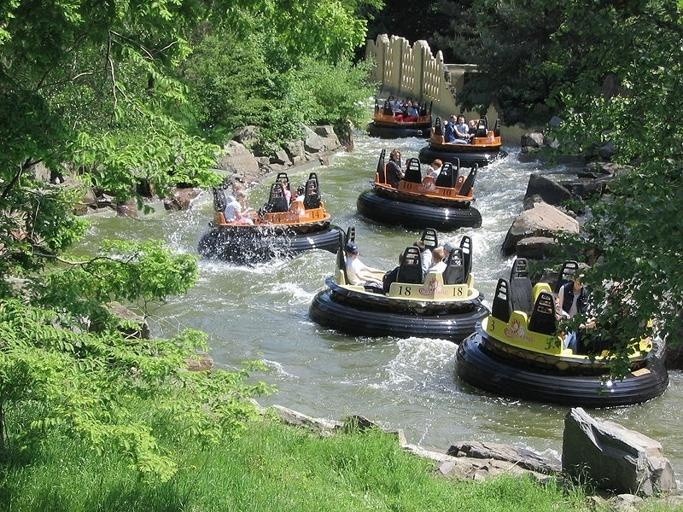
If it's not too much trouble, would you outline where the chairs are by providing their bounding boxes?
[375,96,433,116]
[493,256,593,335]
[336,227,472,291]
[377,149,479,195]
[434,115,501,138]
[213,172,320,216]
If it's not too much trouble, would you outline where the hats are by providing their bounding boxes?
[442,242,456,252]
[345,243,358,252]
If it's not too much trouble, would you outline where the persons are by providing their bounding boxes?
[225,180,316,226]
[382,239,461,292]
[544,271,639,356]
[345,241,390,295]
[382,97,495,194]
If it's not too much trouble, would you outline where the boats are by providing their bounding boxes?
[372,97,434,129]
[419,116,508,170]
[200,171,348,271]
[357,148,482,235]
[308,225,490,345]
[457,259,670,409]
